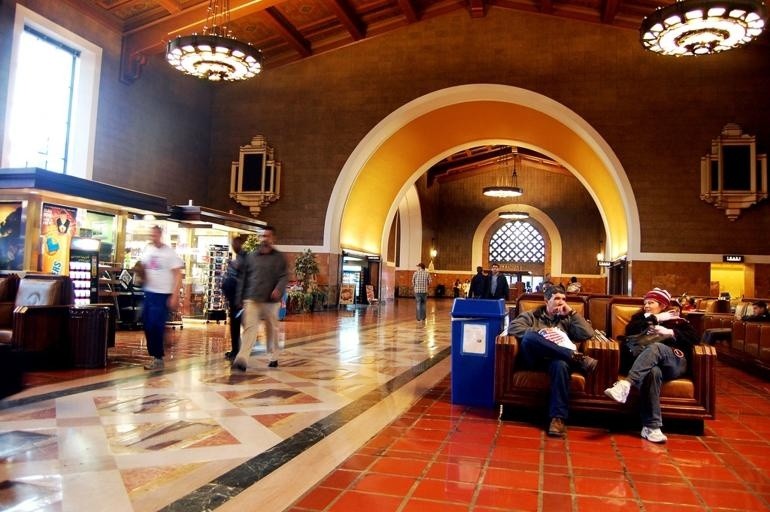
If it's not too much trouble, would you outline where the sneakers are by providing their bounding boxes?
[604,380,630,404]
[641,427,667,443]
[144,358,164,370]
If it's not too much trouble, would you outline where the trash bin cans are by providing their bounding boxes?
[70,307,110,370]
[450,297,511,407]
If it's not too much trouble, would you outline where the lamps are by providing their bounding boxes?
[499,153,529,220]
[639,0,770,57]
[483,144,523,197]
[165,0,265,83]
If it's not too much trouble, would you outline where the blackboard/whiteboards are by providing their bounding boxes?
[365,284,375,302]
[338,282,356,306]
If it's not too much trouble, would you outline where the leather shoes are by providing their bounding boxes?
[548,418,564,436]
[572,351,599,373]
[231,364,246,372]
[269,361,278,367]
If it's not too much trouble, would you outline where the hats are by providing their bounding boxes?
[644,287,671,307]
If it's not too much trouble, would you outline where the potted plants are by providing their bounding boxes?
[286,248,329,314]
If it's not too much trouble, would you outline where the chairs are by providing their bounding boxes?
[0,274,117,353]
[492,293,770,435]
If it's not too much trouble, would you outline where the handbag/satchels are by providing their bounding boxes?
[625,330,672,357]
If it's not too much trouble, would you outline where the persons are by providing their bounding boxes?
[600,288,697,442]
[131,225,185,372]
[222,233,247,359]
[508,287,602,436]
[452,263,581,301]
[699,301,770,346]
[412,262,433,322]
[230,225,288,374]
